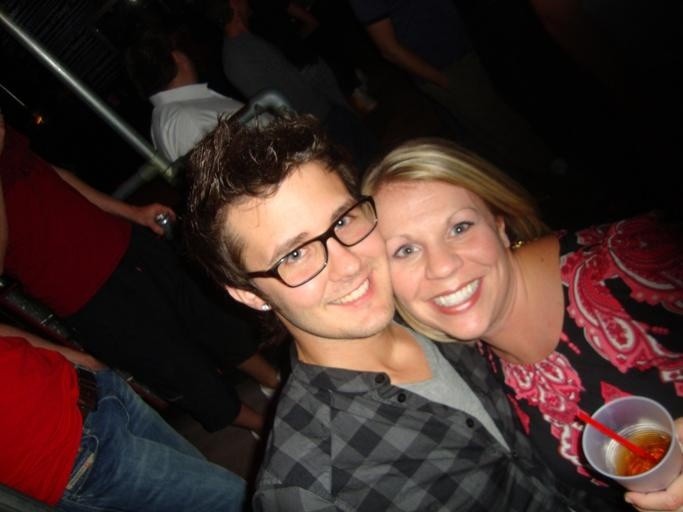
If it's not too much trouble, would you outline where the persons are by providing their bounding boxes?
[361,136,682,512]
[0,325,251,512]
[149,0,561,171]
[0,132,281,431]
[182,105,586,512]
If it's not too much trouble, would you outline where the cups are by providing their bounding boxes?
[581,396,683,491]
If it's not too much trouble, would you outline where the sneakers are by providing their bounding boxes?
[258,374,283,399]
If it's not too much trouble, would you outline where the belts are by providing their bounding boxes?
[70,367,98,419]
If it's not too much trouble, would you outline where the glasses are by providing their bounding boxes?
[246,196,378,288]
